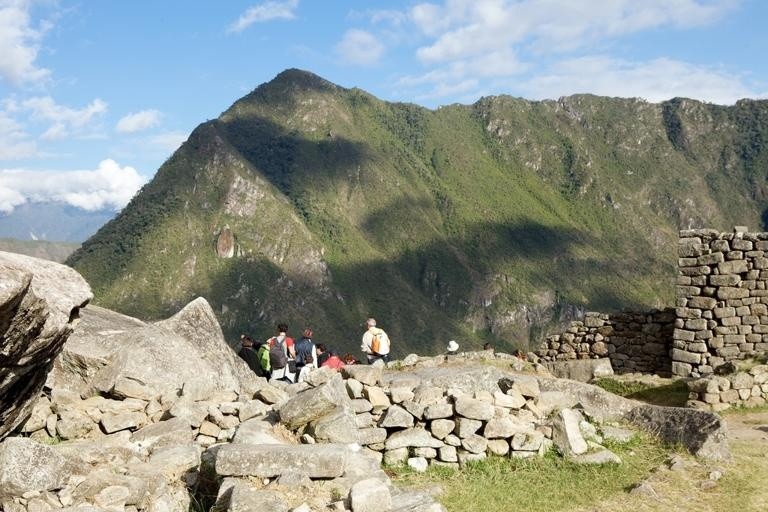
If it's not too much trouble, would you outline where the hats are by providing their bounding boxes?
[447,340,459,352]
[304,328,313,338]
[367,319,376,326]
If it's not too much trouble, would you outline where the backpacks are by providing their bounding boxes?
[258,336,288,372]
[368,328,390,357]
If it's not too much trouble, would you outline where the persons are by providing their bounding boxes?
[238,323,360,384]
[360,317,390,365]
[484,342,491,350]
[443,341,460,357]
[512,348,526,359]
